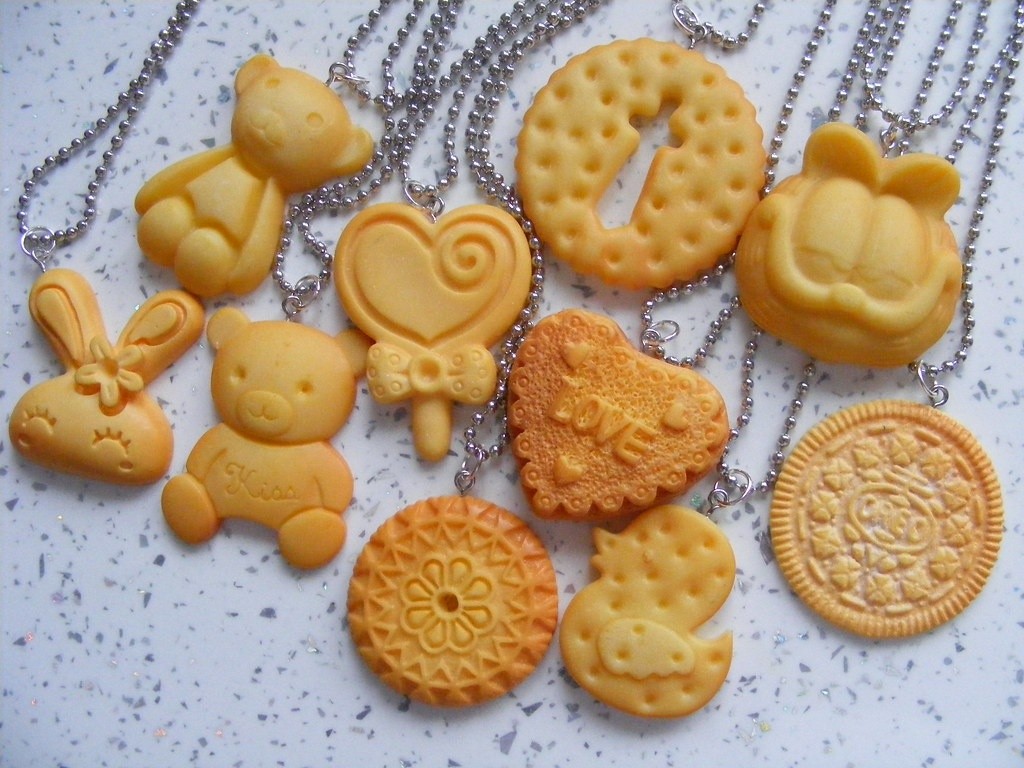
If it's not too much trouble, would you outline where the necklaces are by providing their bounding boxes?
[638,0,1024,520]
[272,0,600,498]
[17,0,197,273]
